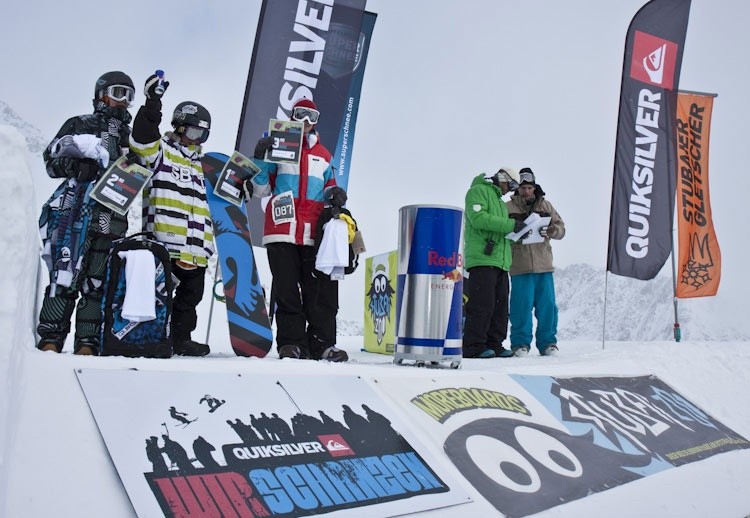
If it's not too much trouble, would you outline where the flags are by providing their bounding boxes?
[667,93,722,298]
[605,0,692,280]
[235,0,378,251]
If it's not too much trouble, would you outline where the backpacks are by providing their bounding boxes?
[99,231,172,359]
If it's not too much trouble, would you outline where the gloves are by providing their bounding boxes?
[124,151,140,165]
[144,74,169,101]
[325,187,347,207]
[540,223,555,238]
[255,137,274,159]
[514,220,527,233]
[520,232,529,239]
[73,158,99,183]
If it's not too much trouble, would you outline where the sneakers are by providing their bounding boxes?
[38,336,64,353]
[309,344,348,362]
[74,336,100,356]
[279,344,301,359]
[173,339,210,355]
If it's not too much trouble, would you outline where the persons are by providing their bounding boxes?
[248,99,349,362]
[35,71,135,356]
[462,164,532,358]
[127,69,254,359]
[505,167,566,358]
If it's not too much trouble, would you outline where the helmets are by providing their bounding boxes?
[95,71,135,92]
[171,101,211,129]
[500,166,520,184]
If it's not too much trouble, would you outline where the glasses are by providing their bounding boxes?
[292,106,321,124]
[108,85,134,107]
[185,125,209,143]
[508,180,519,191]
[519,173,533,184]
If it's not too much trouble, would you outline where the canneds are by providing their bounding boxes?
[394,204,463,362]
[262,130,268,138]
[155,70,165,94]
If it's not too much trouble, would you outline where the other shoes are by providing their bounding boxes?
[479,349,496,358]
[497,350,513,357]
[514,347,528,357]
[544,347,559,356]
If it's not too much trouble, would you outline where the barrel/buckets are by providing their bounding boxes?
[393,203,466,369]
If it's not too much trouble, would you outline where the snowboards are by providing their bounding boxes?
[201,150,273,357]
